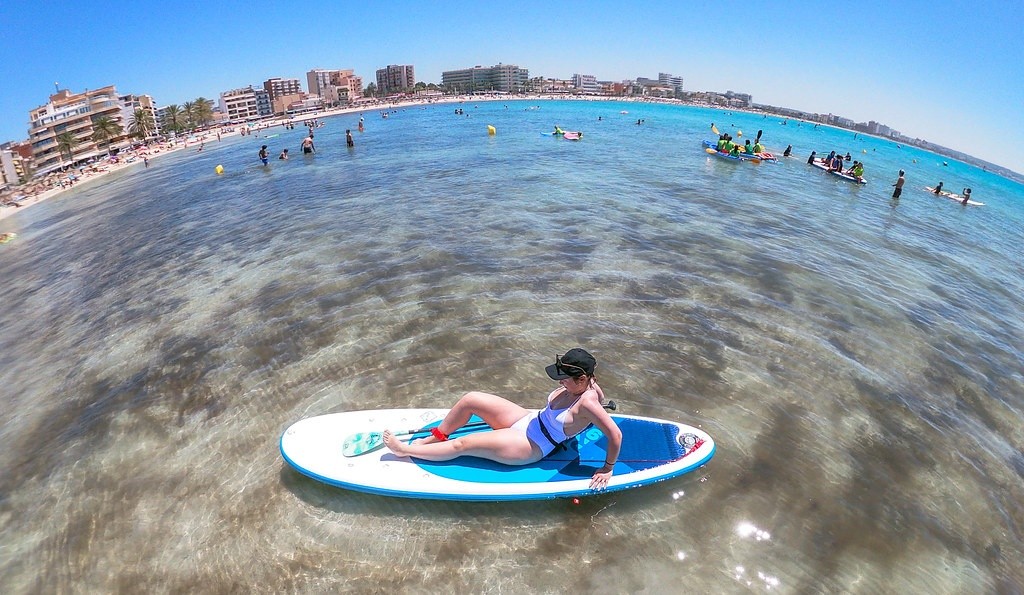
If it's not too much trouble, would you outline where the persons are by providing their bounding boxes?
[553,116,643,140]
[711,111,802,159]
[384,347,623,492]
[12,129,211,208]
[379,102,540,118]
[807,133,971,205]
[216,113,366,165]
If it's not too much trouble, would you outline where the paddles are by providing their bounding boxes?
[710,122,721,136]
[706,148,722,154]
[342,399,618,458]
[757,129,762,140]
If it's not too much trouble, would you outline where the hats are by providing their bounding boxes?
[545,348,596,380]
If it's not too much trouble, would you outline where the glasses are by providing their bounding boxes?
[556,354,587,374]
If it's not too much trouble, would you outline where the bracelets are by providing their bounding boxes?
[606,461,615,465]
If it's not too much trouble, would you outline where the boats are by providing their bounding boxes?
[540,130,580,140]
[701,140,771,162]
[813,158,869,185]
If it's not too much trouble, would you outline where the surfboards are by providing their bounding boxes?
[564,131,581,140]
[539,131,564,137]
[280,407,718,506]
